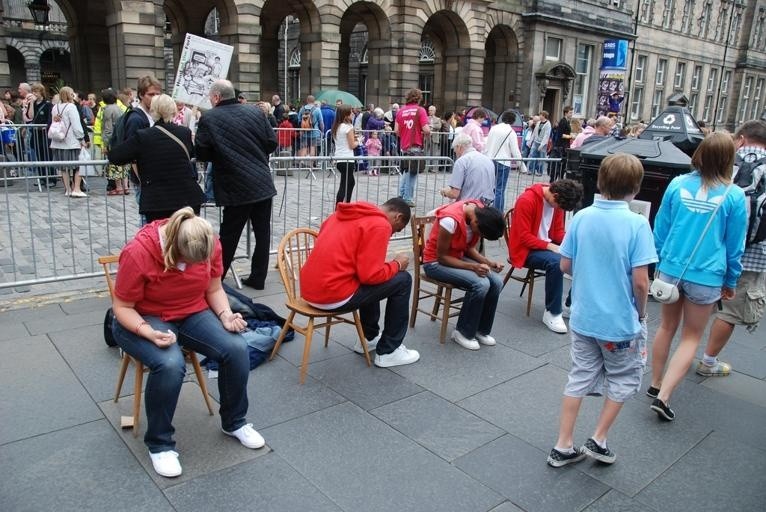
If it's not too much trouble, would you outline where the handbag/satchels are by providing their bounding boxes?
[2,130,16,143]
[404,147,426,175]
[103,308,118,348]
[650,278,680,305]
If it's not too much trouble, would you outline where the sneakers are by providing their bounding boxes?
[562,302,571,319]
[221,420,266,450]
[402,198,417,208]
[430,169,438,172]
[646,386,661,398]
[374,343,420,368]
[695,360,732,378]
[526,170,544,177]
[546,446,586,468]
[367,171,378,176]
[201,197,217,207]
[649,397,675,421]
[66,183,90,198]
[542,308,567,334]
[580,436,617,465]
[34,178,57,188]
[474,331,497,347]
[148,442,184,479]
[354,333,382,354]
[450,328,481,351]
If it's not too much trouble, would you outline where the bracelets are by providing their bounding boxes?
[215,309,234,321]
[133,321,150,337]
[639,312,647,322]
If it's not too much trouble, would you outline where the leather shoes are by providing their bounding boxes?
[239,277,265,290]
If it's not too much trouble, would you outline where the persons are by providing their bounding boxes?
[417,197,508,350]
[181,54,225,80]
[108,204,269,480]
[297,195,425,369]
[694,118,765,379]
[505,177,589,336]
[194,78,279,291]
[2,75,711,224]
[545,151,664,469]
[647,125,749,422]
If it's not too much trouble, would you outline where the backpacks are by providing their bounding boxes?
[109,102,150,150]
[300,104,318,131]
[731,150,766,248]
[48,101,71,141]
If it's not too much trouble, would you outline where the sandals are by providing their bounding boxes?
[106,188,131,196]
